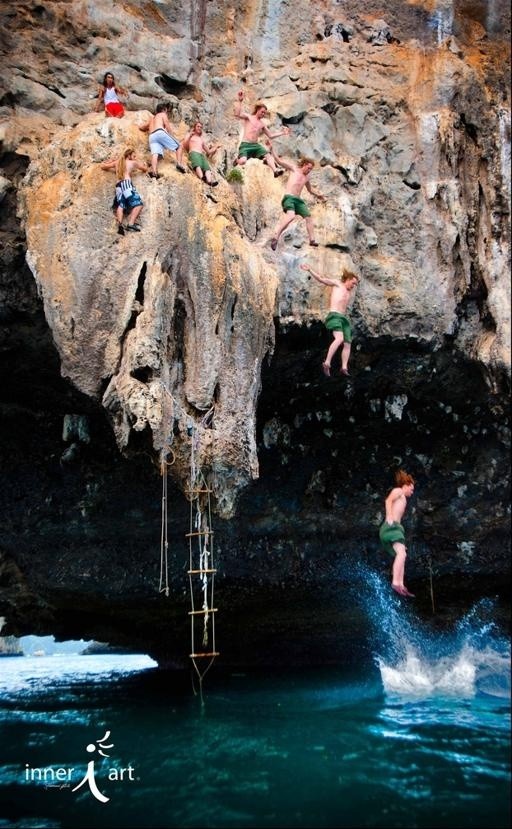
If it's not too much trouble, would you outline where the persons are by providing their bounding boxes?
[265,138,326,251]
[139,103,185,178]
[180,121,221,186]
[380,470,416,597]
[100,148,147,234]
[94,73,130,119]
[233,90,290,177]
[298,263,360,377]
